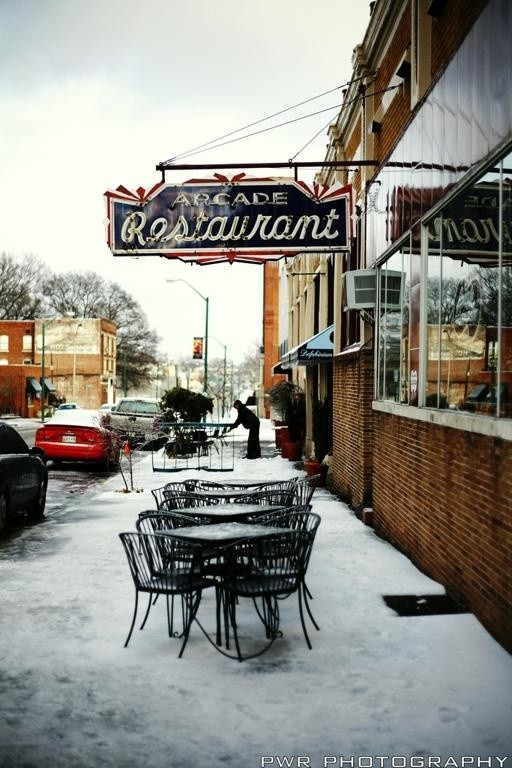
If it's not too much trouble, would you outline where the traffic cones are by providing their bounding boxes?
[124,440,130,456]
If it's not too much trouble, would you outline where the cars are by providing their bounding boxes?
[53,403,81,416]
[0,421,47,540]
[107,396,177,451]
[98,403,119,412]
[36,409,128,474]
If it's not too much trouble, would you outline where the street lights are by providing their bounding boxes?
[41,312,76,422]
[166,277,208,396]
[204,335,226,419]
[122,346,143,398]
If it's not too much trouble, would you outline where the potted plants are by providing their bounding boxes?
[160,385,197,457]
[302,394,322,478]
[194,392,215,441]
[268,380,306,460]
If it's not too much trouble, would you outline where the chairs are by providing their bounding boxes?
[118,473,321,660]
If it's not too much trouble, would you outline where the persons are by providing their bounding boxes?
[227,400,262,459]
